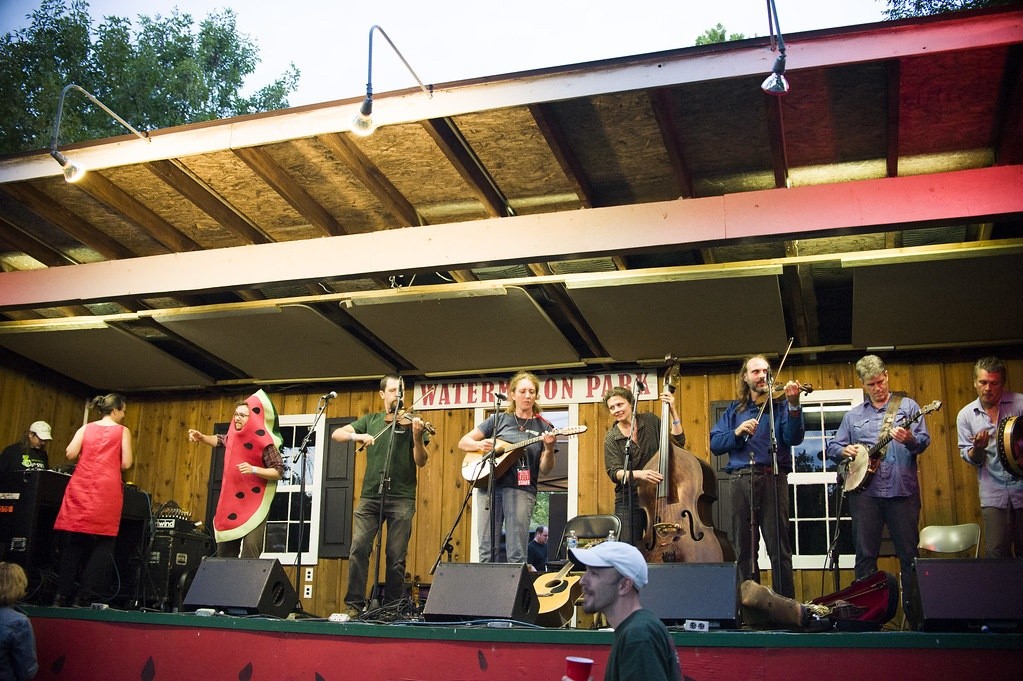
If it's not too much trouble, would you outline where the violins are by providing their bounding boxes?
[384,408,436,435]
[754,381,814,411]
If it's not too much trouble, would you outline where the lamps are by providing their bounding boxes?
[51,84,149,183]
[760,0,789,94]
[346,24,432,136]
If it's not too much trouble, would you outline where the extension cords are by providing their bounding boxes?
[194,608,215,617]
[486,621,514,628]
[91,602,109,610]
[328,613,350,622]
[685,620,709,631]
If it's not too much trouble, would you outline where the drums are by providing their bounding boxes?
[996,416,1023,480]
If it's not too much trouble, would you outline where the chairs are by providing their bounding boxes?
[900,523,982,631]
[545,514,622,628]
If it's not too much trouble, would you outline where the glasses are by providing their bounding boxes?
[232,411,249,419]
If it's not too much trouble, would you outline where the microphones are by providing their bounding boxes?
[399,377,403,400]
[766,369,773,382]
[636,378,644,391]
[321,390,338,398]
[494,393,508,401]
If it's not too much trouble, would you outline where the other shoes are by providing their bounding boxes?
[910,623,924,631]
[343,604,360,619]
[72,595,92,608]
[51,593,69,609]
[384,611,398,621]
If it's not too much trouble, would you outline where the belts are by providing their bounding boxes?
[731,466,773,475]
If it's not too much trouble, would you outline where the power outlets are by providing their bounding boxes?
[304,568,313,582]
[304,585,312,598]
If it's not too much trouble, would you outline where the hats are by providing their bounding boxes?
[567,541,648,593]
[30,421,54,441]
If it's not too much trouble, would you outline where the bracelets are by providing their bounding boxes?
[789,404,801,411]
[252,465,256,474]
[672,419,680,424]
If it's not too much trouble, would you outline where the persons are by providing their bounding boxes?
[826,354,930,632]
[527,525,549,572]
[0,421,54,471]
[188,388,286,559]
[568,541,683,681]
[955,357,1023,557]
[51,393,133,609]
[602,386,685,547]
[457,371,556,564]
[0,561,39,681]
[710,353,805,600]
[329,372,430,622]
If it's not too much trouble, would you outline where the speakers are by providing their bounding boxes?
[638,562,745,629]
[422,562,540,627]
[902,556,1023,632]
[136,528,213,610]
[182,556,299,618]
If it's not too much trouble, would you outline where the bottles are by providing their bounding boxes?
[608,530,615,542]
[567,530,579,548]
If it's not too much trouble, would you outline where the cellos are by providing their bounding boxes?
[632,347,738,564]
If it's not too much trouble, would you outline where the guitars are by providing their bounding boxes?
[461,424,588,488]
[531,538,605,628]
[836,400,943,498]
